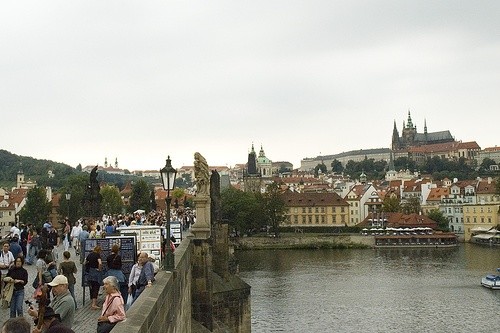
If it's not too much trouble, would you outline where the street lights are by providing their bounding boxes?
[160,154,178,271]
[65,189,72,218]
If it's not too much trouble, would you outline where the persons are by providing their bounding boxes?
[63,209,196,252]
[4,221,60,265]
[32,250,78,311]
[269,234,276,240]
[97,276,126,333]
[0,242,31,333]
[26,275,76,333]
[129,253,155,306]
[86,245,125,309]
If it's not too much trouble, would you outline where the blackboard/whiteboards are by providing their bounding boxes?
[81,237,137,287]
[116,226,161,269]
[161,222,182,245]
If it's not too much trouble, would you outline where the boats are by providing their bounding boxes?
[481,267,500,289]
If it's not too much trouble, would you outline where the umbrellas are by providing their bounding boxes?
[362,227,432,233]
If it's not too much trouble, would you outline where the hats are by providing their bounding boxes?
[34,306,61,326]
[47,275,68,286]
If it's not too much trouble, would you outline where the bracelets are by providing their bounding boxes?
[148,281,152,284]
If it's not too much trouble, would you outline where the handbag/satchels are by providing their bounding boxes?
[97,296,120,333]
